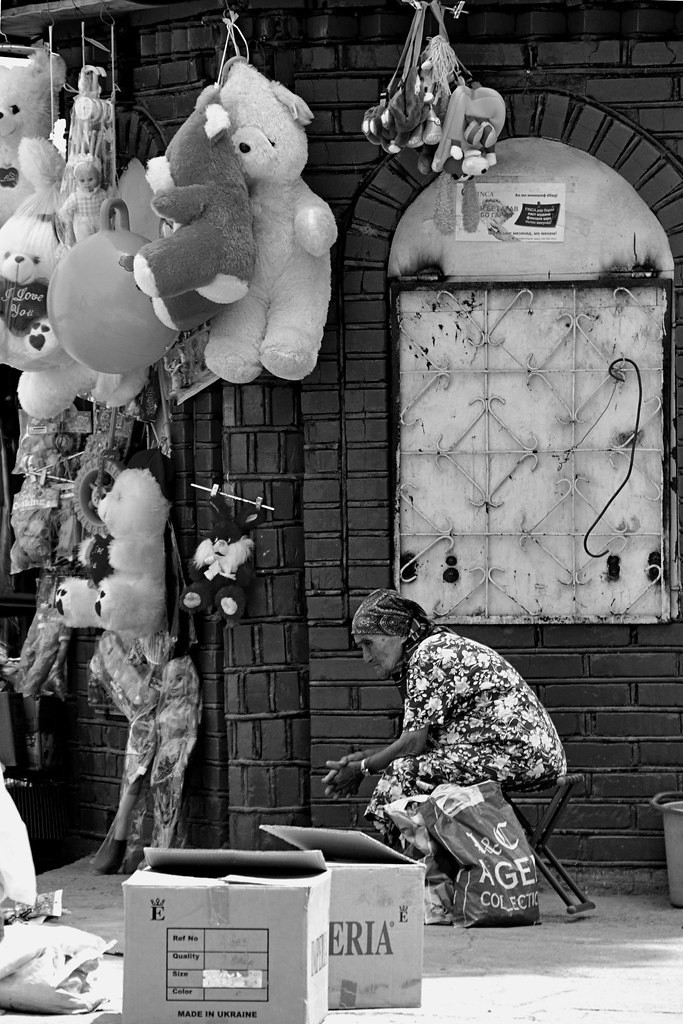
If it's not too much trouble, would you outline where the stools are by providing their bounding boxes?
[498,772,597,916]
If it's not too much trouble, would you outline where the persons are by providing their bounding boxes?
[138,663,198,869]
[61,154,117,241]
[92,672,161,875]
[321,587,566,924]
[488,219,521,241]
[15,586,69,702]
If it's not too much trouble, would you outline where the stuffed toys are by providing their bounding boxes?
[0,52,338,421]
[361,63,506,234]
[54,467,171,636]
[179,493,266,621]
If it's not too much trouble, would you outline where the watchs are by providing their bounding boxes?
[360,758,371,777]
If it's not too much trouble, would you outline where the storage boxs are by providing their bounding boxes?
[260,824,427,1012]
[122,843,330,1024]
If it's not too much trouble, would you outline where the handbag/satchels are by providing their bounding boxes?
[417,780,543,926]
[382,795,453,925]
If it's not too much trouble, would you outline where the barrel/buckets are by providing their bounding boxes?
[650,791,683,909]
